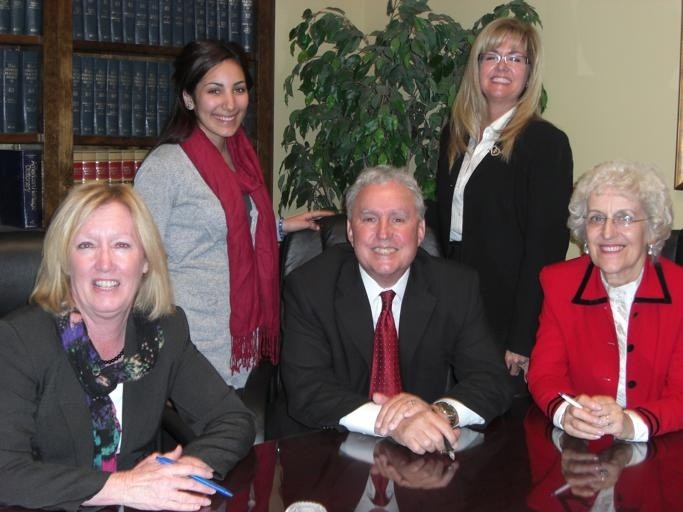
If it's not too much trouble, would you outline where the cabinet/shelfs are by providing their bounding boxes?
[0,0,279,253]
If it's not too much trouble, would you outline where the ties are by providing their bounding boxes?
[369,290,403,402]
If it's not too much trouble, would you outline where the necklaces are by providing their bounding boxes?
[101,348,126,367]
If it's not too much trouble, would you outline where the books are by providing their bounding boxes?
[71,52,256,141]
[73,1,257,53]
[73,145,152,188]
[0,2,45,228]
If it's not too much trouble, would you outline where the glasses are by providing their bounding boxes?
[583,215,648,227]
[479,52,530,65]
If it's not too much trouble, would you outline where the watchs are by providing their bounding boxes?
[433,399,459,430]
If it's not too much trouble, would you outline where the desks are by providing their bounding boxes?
[0,388,682,511]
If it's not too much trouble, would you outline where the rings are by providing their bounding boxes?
[606,414,612,425]
[599,470,609,484]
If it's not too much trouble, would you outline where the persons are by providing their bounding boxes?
[418,16,574,382]
[519,413,683,511]
[528,164,681,438]
[130,37,334,453]
[274,166,520,460]
[278,425,530,511]
[0,182,257,510]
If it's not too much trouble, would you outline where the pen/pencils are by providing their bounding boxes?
[156,456,234,498]
[431,408,456,461]
[550,483,571,497]
[557,391,583,409]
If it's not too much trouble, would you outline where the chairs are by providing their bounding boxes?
[258,199,451,440]
[0,243,48,322]
[654,228,681,269]
